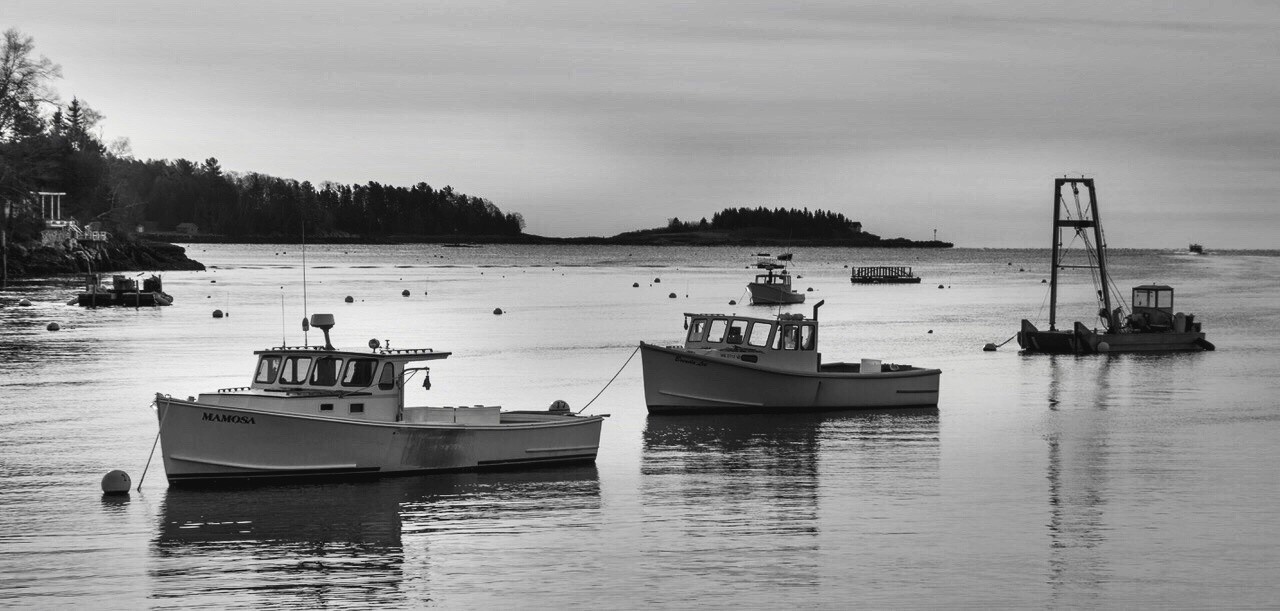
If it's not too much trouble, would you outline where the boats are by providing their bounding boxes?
[1017,173,1215,355]
[149,219,611,494]
[641,299,942,416]
[747,238,805,306]
